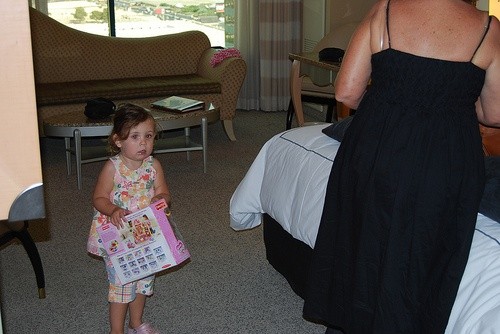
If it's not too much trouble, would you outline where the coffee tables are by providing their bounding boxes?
[42,105,210,187]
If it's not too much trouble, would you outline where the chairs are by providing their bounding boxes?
[286,89,333,130]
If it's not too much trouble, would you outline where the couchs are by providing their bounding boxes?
[29,6,247,142]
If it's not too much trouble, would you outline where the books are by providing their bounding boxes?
[151,95,205,113]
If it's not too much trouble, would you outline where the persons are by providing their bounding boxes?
[87,104,171,334]
[302,0,500,334]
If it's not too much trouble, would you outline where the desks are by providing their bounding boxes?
[288,52,352,127]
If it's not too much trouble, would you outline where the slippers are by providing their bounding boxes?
[127,322,159,334]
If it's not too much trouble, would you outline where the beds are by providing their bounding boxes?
[228,122,500,334]
